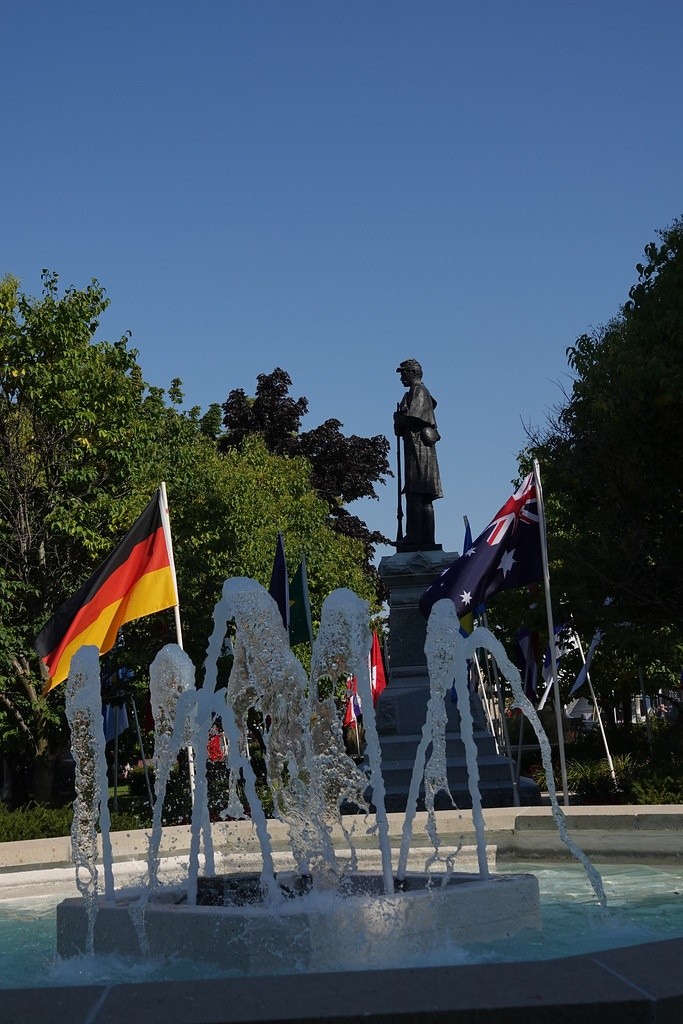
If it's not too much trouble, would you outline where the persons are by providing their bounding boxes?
[392,360,443,547]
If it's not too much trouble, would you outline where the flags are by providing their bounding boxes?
[32,488,179,693]
[268,540,388,728]
[418,471,563,708]
